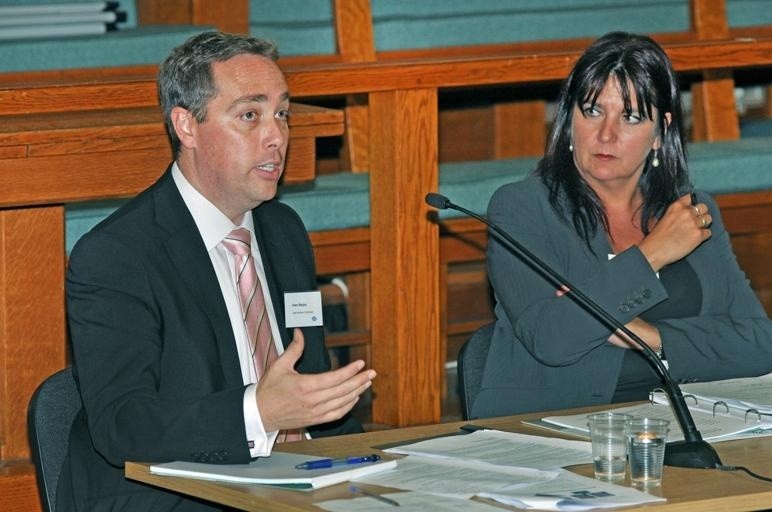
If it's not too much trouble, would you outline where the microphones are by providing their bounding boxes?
[425,192,723,469]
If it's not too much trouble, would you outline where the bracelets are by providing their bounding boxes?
[654,329,664,359]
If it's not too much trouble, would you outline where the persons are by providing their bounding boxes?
[55,31,378,511]
[467,30,772,419]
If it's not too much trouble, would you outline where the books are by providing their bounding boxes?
[521,372,772,445]
[149,449,399,490]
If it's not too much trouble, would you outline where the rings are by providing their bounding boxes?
[701,217,708,228]
[691,206,700,215]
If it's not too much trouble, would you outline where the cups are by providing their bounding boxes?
[587,412,633,480]
[623,418,669,489]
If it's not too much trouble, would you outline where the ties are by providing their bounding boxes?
[220,227,302,446]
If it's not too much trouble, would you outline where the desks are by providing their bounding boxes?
[125,373,771,512]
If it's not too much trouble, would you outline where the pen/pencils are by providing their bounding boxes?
[350,486,399,507]
[296,453,381,471]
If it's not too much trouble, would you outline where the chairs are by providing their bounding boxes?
[27,366,86,511]
